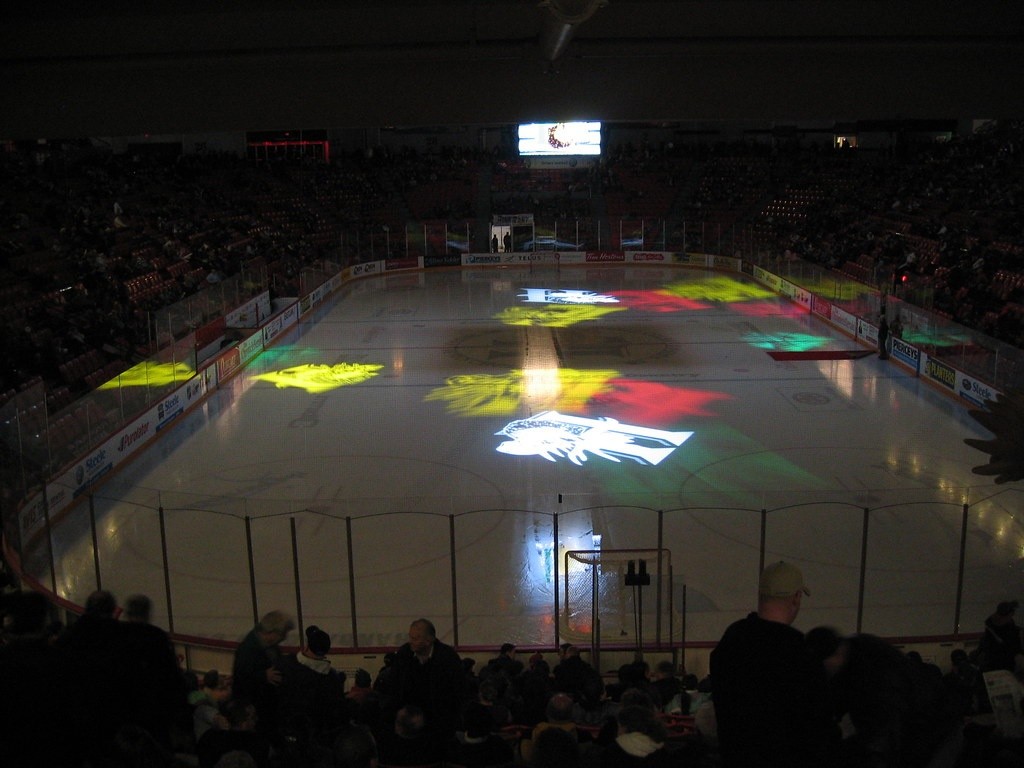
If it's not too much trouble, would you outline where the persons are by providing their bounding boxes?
[0,561,1024,768]
[0,121,1024,552]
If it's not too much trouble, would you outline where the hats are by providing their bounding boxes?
[127,591,161,609]
[304,626,333,658]
[760,559,812,598]
[951,648,968,661]
[996,601,1021,617]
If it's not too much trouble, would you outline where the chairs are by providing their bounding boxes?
[1,149,1024,457]
[500,714,698,744]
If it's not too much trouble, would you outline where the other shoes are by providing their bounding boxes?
[879,354,882,358]
[880,357,888,360]
